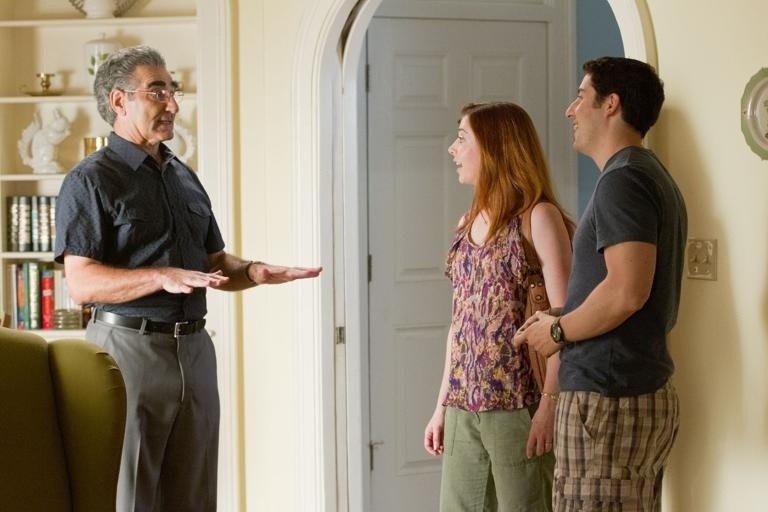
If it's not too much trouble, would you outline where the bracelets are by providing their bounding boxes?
[539,392,557,401]
[244,260,267,284]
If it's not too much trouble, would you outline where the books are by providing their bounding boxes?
[5,194,94,330]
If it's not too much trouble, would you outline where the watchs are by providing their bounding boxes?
[549,316,575,348]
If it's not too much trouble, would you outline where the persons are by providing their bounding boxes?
[509,54,694,512]
[53,44,325,512]
[424,100,578,512]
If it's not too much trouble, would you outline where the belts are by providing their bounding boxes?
[92,307,208,338]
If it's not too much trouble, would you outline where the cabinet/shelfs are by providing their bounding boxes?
[1,16,198,352]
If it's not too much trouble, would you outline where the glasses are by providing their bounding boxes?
[120,83,184,100]
